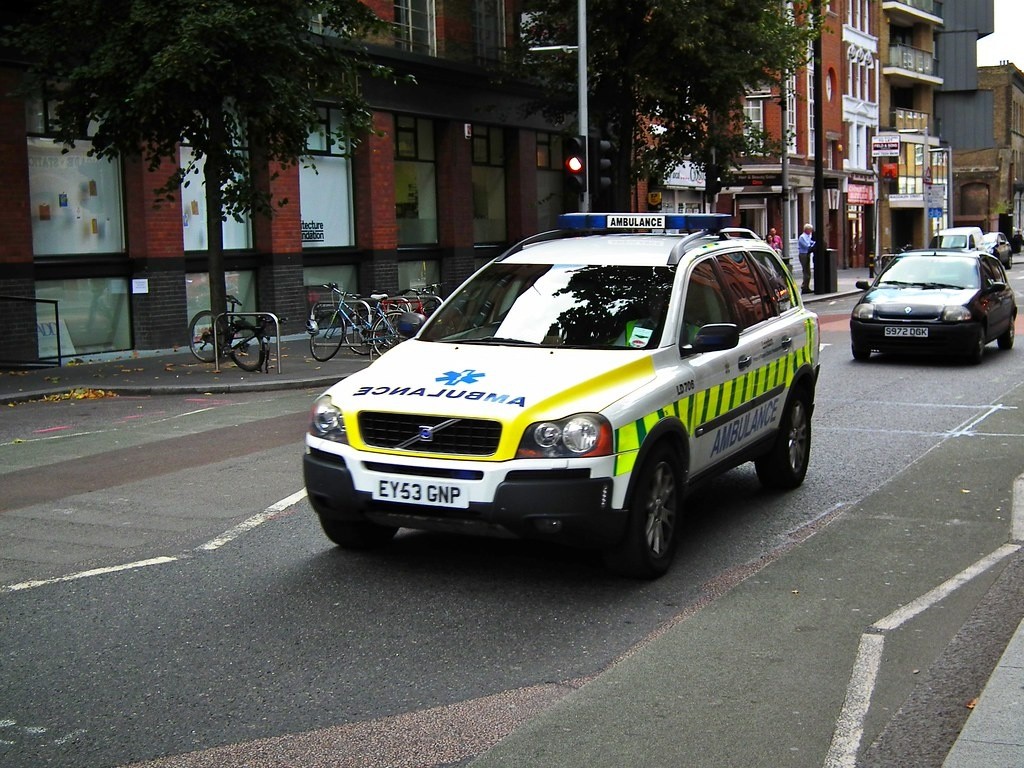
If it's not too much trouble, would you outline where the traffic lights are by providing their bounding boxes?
[560,133,586,195]
[588,128,612,200]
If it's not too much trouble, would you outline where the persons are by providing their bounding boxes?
[612,282,701,353]
[766,227,784,254]
[797,223,818,295]
[765,234,778,251]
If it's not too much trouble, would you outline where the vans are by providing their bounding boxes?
[930,226,987,256]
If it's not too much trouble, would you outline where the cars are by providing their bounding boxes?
[982,232,1013,270]
[849,245,1018,365]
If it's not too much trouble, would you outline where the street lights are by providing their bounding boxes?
[746,93,792,274]
[928,145,954,229]
[899,126,932,251]
[529,44,591,214]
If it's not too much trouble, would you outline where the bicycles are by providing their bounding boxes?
[187,294,288,374]
[309,278,468,362]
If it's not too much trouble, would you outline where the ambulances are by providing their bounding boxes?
[300,211,821,581]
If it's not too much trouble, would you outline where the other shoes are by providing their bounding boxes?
[802,289,814,294]
